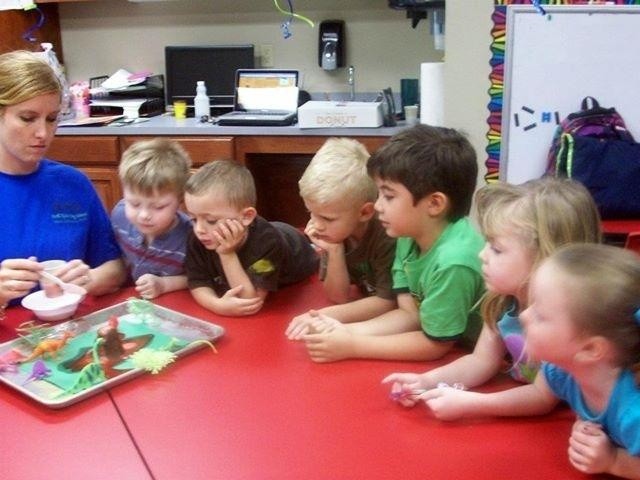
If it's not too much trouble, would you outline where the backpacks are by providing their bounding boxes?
[544,96,634,179]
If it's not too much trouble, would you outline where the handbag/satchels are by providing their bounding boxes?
[556,124,640,219]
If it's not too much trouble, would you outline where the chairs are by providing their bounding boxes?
[623,232,638,254]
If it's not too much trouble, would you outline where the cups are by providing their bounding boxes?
[175,100,185,119]
[404,105,417,124]
[38,261,70,299]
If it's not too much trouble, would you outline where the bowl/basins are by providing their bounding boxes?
[23,289,85,321]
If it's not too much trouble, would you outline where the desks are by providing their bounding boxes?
[0,268,638,478]
[597,219,640,246]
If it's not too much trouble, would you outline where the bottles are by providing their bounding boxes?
[194,81,210,118]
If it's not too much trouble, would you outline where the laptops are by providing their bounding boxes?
[216,69,299,126]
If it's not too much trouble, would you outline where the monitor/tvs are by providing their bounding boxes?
[163,45,254,118]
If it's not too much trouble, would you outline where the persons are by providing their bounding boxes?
[297,124,493,366]
[109,137,202,300]
[1,49,128,313]
[381,175,602,426]
[514,242,640,480]
[182,158,321,318]
[282,136,400,342]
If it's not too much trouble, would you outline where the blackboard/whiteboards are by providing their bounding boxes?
[498,4,640,185]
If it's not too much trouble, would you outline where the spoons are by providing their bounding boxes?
[33,266,80,296]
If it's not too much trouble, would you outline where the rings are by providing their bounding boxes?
[78,274,93,284]
[8,280,16,292]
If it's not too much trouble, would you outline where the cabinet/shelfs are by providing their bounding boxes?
[120,133,236,216]
[44,135,120,217]
